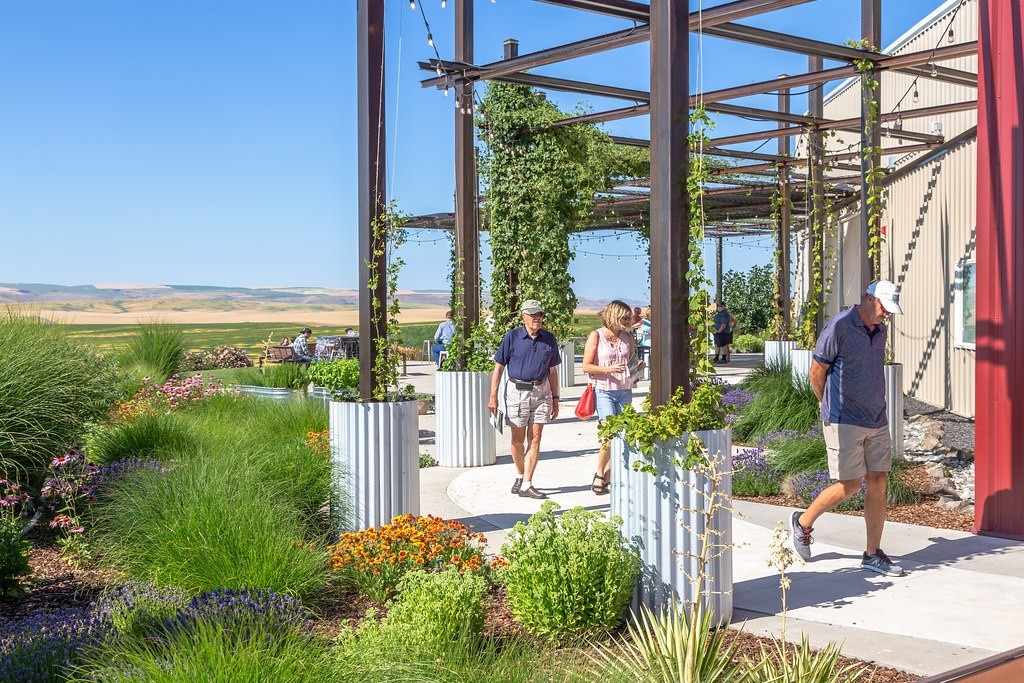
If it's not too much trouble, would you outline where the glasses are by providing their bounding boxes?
[876,299,891,314]
[525,312,544,318]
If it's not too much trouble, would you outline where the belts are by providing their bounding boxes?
[509,377,548,386]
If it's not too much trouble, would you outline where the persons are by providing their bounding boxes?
[344,327,353,334]
[431,310,456,366]
[279,327,315,363]
[787,278,906,577]
[487,299,563,499]
[632,304,652,382]
[583,299,646,494]
[630,307,643,329]
[711,301,736,363]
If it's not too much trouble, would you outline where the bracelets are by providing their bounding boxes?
[552,396,559,400]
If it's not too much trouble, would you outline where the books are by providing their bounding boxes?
[627,352,644,386]
[489,409,504,435]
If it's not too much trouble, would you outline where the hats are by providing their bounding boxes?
[866,280,903,314]
[716,302,725,306]
[520,299,545,316]
[299,328,312,334]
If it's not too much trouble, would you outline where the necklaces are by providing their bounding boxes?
[606,329,612,342]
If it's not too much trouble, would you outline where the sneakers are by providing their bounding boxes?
[860,548,904,576]
[789,510,815,561]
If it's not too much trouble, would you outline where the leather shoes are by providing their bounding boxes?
[517,486,546,499]
[510,477,524,494]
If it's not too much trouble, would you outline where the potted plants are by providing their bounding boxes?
[326,199,416,540]
[841,39,906,469]
[764,175,803,371]
[610,109,737,634]
[551,335,576,389]
[792,125,829,392]
[434,188,498,466]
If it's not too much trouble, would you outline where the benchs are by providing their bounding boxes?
[259,343,315,375]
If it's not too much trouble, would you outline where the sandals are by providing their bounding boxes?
[591,472,605,495]
[604,482,610,493]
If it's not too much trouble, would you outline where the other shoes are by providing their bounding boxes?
[711,359,719,363]
[719,359,728,363]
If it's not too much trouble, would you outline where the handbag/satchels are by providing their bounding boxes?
[574,383,596,420]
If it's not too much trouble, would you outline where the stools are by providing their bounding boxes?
[634,346,651,383]
[422,339,435,364]
[439,351,450,370]
